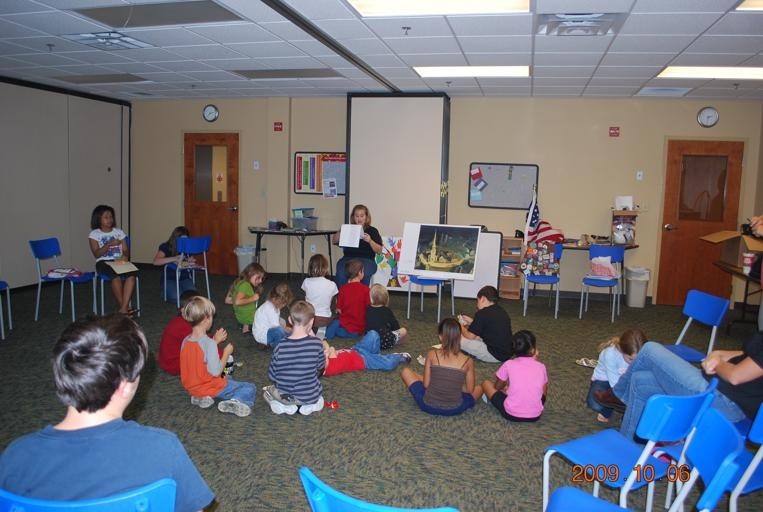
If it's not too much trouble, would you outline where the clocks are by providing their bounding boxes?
[697,106,720,127]
[202,104,219,123]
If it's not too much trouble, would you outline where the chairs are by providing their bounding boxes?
[0,478,177,512]
[523,244,564,319]
[162,234,214,309]
[0,281,13,340]
[93,236,141,317]
[297,465,460,512]
[406,275,455,324]
[579,244,625,324]
[28,237,96,324]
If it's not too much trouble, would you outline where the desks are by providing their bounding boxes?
[248,225,338,287]
[532,238,639,322]
[713,261,763,335]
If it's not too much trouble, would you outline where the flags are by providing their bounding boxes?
[523,189,565,248]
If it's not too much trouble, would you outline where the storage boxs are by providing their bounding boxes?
[610,210,639,243]
[500,259,520,277]
[699,230,763,268]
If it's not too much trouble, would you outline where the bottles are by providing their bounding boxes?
[226,352,234,374]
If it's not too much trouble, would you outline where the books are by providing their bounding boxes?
[103,259,139,274]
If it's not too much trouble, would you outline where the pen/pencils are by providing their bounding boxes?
[746,217,753,223]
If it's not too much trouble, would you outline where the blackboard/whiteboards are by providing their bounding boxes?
[452,231,502,302]
[295,152,346,195]
[468,162,539,210]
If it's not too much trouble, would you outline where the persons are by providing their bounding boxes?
[321,258,370,342]
[320,327,411,378]
[87,203,143,320]
[591,325,762,442]
[454,284,515,365]
[151,225,201,306]
[328,201,384,288]
[748,213,763,331]
[584,326,648,424]
[176,293,259,418]
[248,280,294,349]
[398,314,485,417]
[480,327,550,424]
[260,297,327,415]
[0,307,220,512]
[224,262,264,335]
[155,289,223,374]
[297,252,339,330]
[363,283,408,351]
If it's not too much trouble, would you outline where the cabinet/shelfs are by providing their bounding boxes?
[498,236,524,300]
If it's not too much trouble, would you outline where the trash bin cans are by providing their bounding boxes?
[235,247,255,277]
[626,267,651,308]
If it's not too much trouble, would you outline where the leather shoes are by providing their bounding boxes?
[592,387,626,413]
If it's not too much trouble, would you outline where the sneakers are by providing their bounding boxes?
[190,395,215,409]
[298,395,325,416]
[392,352,412,364]
[217,399,252,418]
[325,319,340,340]
[256,344,267,351]
[336,328,360,339]
[270,399,299,416]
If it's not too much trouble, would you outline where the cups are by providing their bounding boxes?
[741,223,749,232]
[742,253,753,275]
[268,220,280,231]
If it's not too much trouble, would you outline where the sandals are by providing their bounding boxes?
[575,356,600,368]
[116,308,139,319]
